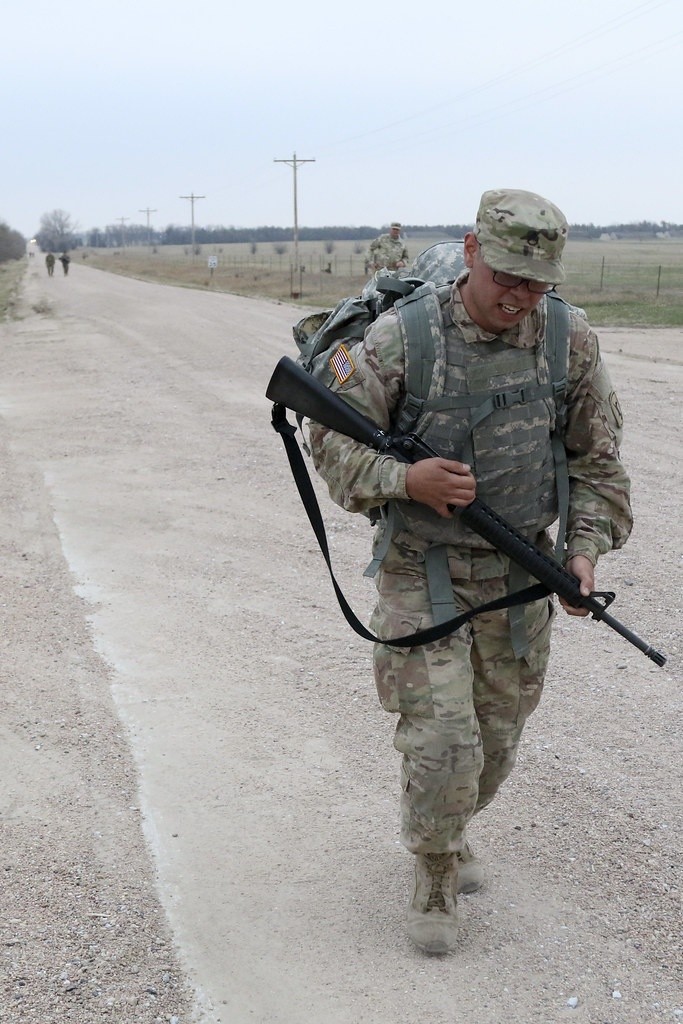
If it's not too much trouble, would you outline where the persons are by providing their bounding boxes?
[29,252,35,257]
[363,222,409,279]
[46,251,55,276]
[301,190,634,953]
[59,250,71,276]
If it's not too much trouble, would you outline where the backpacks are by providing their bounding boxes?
[293,240,569,526]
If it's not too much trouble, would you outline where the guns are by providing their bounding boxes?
[265,353,668,671]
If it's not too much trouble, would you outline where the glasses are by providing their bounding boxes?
[493,270,557,293]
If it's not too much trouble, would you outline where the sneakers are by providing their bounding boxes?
[405,850,458,952]
[457,840,484,894]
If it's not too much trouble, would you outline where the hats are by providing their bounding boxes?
[391,222,401,229]
[473,189,569,282]
